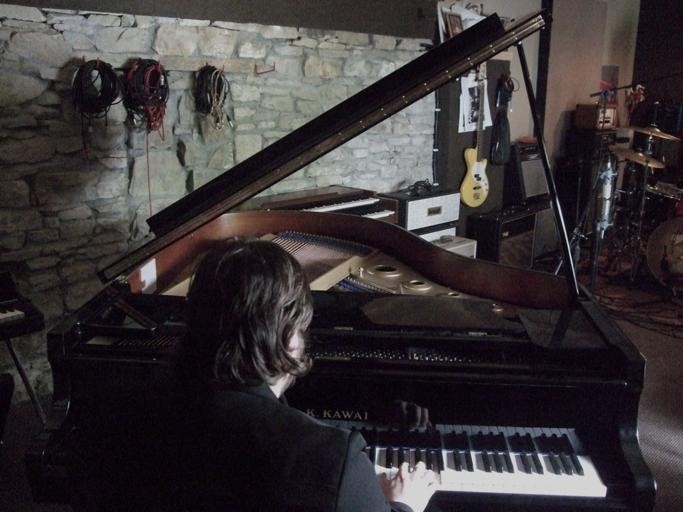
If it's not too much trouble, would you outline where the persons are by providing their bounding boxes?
[42,239,404,512]
[394,399,432,428]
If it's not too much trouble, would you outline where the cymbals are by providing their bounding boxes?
[611,145,665,169]
[626,126,679,141]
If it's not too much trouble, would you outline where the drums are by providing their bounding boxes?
[643,183,681,220]
[646,219,683,291]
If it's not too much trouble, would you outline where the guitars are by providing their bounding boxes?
[460,62,490,208]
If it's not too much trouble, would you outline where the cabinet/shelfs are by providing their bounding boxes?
[377,186,461,244]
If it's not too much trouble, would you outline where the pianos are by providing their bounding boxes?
[25,9,658,512]
[0,295,45,343]
[230,184,400,225]
